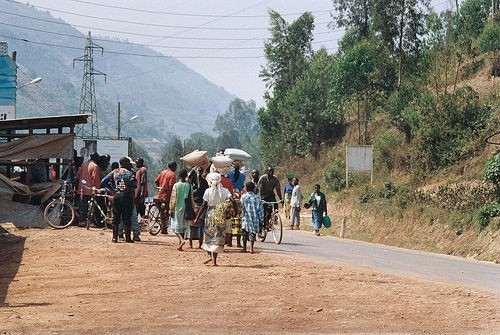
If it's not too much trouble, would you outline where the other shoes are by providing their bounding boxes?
[126,237,134,243]
[119,238,124,241]
[112,238,117,243]
[134,235,141,241]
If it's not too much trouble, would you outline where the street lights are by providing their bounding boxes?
[116,101,139,140]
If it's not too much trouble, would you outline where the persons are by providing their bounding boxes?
[185,165,209,249]
[307,184,327,236]
[257,166,284,237]
[118,157,141,241]
[210,150,225,172]
[101,157,135,243]
[240,182,264,254]
[71,156,84,190]
[194,172,238,266]
[216,168,233,244]
[75,151,101,228]
[225,160,243,248]
[251,169,260,195]
[135,157,147,217]
[168,169,194,251]
[285,177,303,229]
[111,162,119,171]
[282,178,294,220]
[155,161,178,234]
[95,154,111,227]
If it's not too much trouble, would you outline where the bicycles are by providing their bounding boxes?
[258,200,284,244]
[42,180,164,236]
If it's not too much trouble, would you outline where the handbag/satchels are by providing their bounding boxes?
[183,183,196,220]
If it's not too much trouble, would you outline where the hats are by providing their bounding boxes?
[231,161,242,169]
